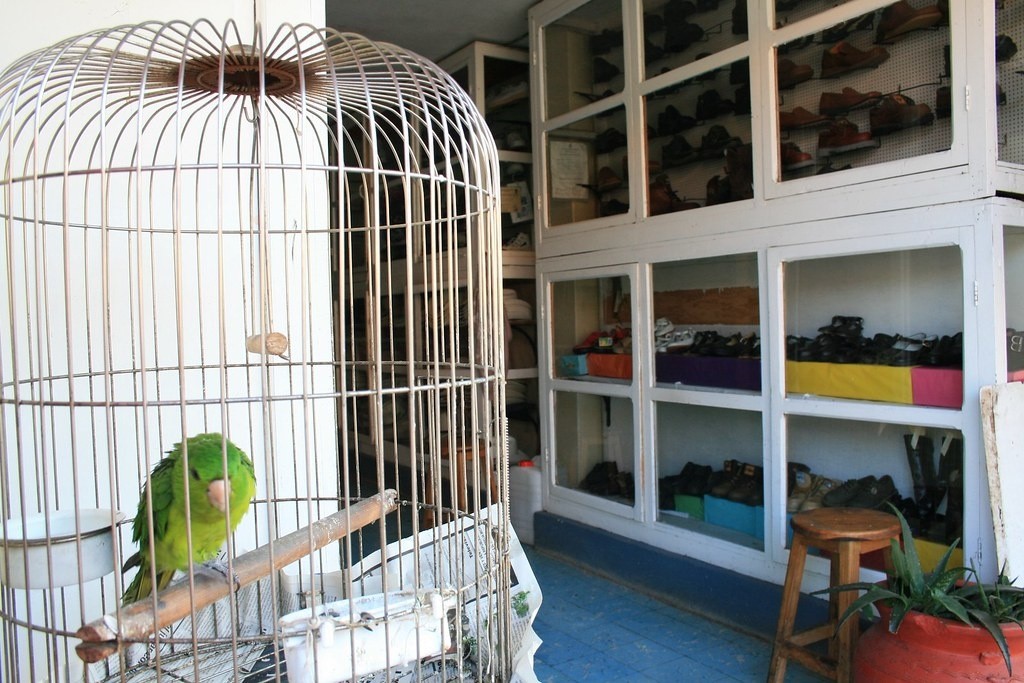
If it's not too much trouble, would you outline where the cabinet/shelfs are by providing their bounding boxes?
[332,38,546,494]
[529,0,1024,260]
[534,196,1024,655]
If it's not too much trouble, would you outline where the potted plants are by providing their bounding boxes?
[809,499,1024,683]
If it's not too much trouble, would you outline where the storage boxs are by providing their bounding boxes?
[562,354,964,409]
[676,495,963,581]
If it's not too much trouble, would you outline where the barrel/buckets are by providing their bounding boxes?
[508,460,543,546]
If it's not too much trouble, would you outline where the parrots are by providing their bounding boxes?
[121,435,257,609]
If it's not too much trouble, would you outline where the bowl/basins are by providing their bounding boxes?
[0,510,127,590]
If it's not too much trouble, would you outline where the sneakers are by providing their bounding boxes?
[502,232,531,251]
[699,125,743,160]
[875,3,942,45]
[867,94,934,135]
[820,41,888,79]
[661,136,698,167]
[703,175,753,206]
[818,122,875,156]
[779,142,812,173]
[776,58,814,89]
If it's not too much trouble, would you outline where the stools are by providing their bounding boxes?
[767,507,903,683]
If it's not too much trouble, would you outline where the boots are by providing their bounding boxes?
[649,173,699,216]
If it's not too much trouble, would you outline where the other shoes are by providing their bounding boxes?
[596,166,622,190]
[586,0,719,64]
[934,0,1004,26]
[591,56,618,83]
[594,128,627,154]
[695,89,735,120]
[599,198,629,217]
[818,86,881,117]
[621,155,659,177]
[936,85,1006,120]
[508,132,524,149]
[822,12,872,43]
[656,105,697,136]
[553,315,964,549]
[943,37,1017,75]
[731,6,747,34]
[778,35,815,55]
[778,105,829,130]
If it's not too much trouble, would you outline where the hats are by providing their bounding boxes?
[345,288,539,466]
